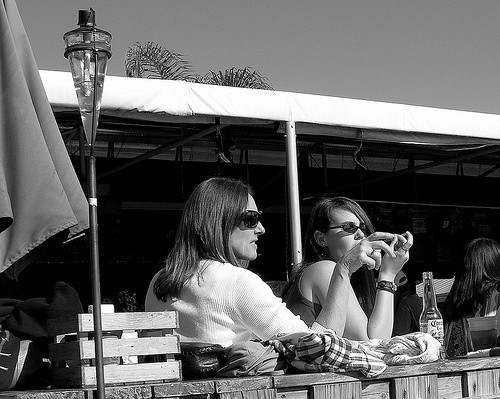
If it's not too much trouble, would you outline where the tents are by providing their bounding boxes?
[39,68,500,281]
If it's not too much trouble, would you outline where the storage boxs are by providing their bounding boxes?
[49,304,182,388]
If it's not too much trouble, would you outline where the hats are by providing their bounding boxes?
[394,270,408,287]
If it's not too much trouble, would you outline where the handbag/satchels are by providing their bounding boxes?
[178,342,227,378]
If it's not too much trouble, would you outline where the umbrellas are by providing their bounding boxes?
[0,1,99,283]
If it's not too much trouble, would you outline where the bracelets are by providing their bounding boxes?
[375,281,397,294]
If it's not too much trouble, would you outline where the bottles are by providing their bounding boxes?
[419,272,446,362]
[87,304,123,367]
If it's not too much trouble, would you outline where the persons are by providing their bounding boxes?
[279,196,500,340]
[145,178,397,347]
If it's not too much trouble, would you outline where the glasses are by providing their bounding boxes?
[328,221,368,234]
[240,210,264,229]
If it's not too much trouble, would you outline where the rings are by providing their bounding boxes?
[402,246,408,253]
[369,250,375,257]
